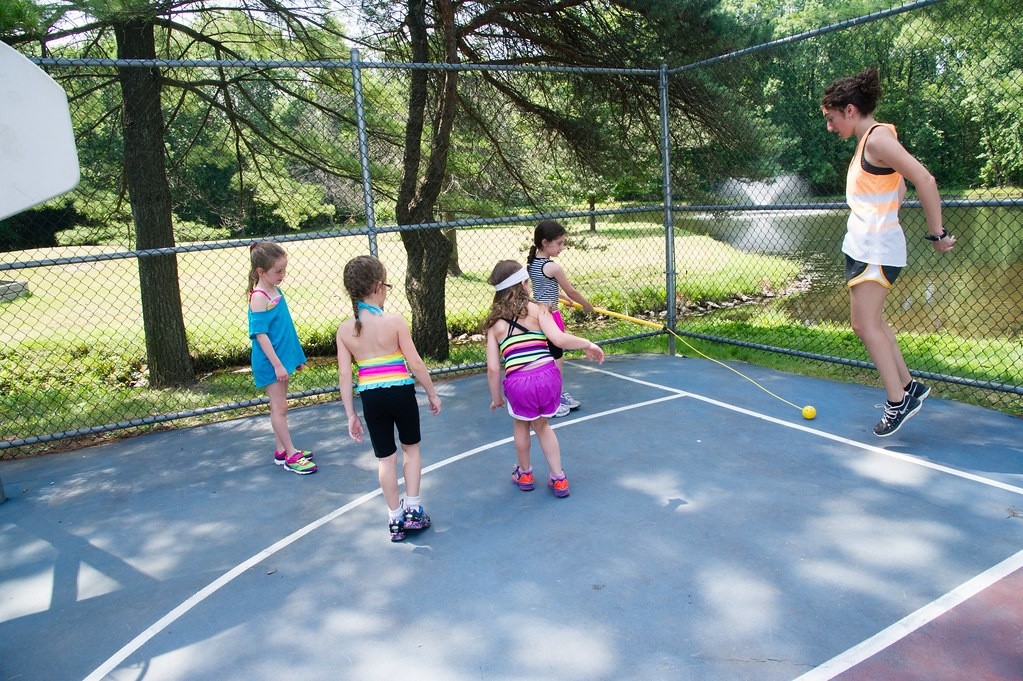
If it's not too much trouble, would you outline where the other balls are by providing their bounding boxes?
[802,406,816,420]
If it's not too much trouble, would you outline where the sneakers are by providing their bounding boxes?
[553,405,570,417]
[547,474,570,497]
[403,504,430,529]
[905,377,933,403]
[872,391,922,438]
[389,517,406,541]
[284,449,318,474]
[560,390,581,407]
[511,463,534,490]
[274,448,313,465]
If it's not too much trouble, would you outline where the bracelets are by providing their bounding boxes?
[925,228,947,241]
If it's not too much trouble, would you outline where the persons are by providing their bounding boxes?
[484,260,604,498]
[820,70,955,438]
[336,255,442,541]
[247,241,318,475]
[526,220,596,418]
